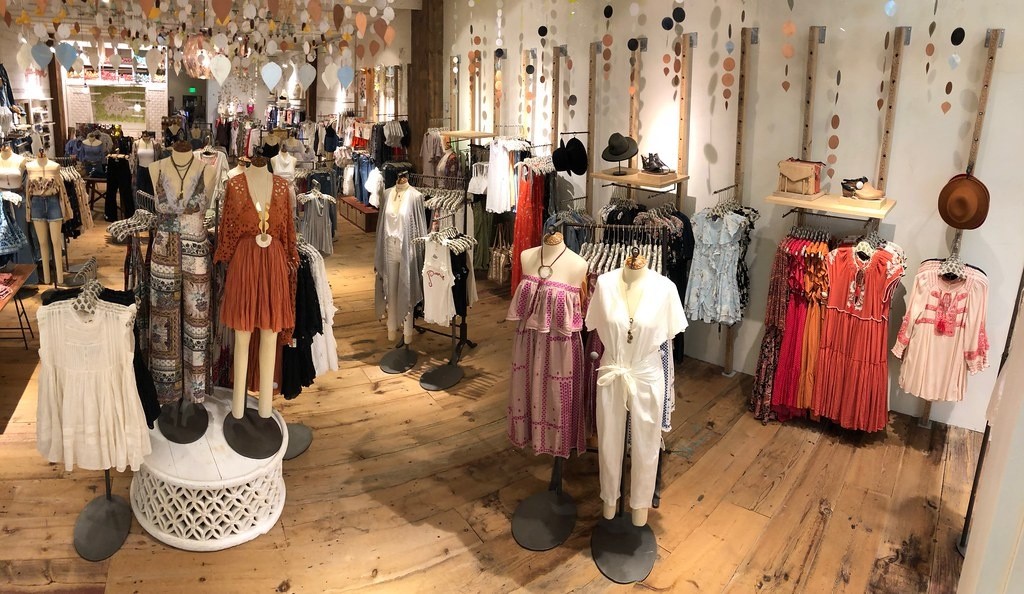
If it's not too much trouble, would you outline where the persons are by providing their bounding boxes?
[584,258,688,525]
[506,231,587,451]
[435,135,457,190]
[0,130,426,437]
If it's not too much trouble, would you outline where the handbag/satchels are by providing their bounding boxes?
[788,157,827,193]
[777,160,815,194]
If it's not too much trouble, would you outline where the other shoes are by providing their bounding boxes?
[654,153,676,173]
[641,153,662,173]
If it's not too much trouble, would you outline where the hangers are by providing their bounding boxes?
[408,174,475,213]
[938,232,966,280]
[611,184,677,216]
[412,227,478,254]
[855,218,882,259]
[294,159,336,207]
[35,259,135,325]
[428,117,450,133]
[563,196,587,218]
[513,142,554,175]
[29,155,83,181]
[703,184,736,218]
[294,216,318,258]
[787,205,833,242]
[105,105,409,158]
[106,189,158,243]
[577,223,661,254]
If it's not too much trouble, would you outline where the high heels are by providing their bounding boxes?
[841,176,884,201]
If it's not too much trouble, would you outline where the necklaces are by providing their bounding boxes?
[439,154,446,165]
[395,188,407,201]
[246,170,274,248]
[170,154,194,167]
[537,244,565,280]
[619,278,645,344]
[171,156,194,200]
[143,138,150,149]
[89,138,96,146]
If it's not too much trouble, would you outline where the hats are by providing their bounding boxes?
[938,173,991,230]
[566,137,587,176]
[601,133,639,163]
[551,138,573,177]
